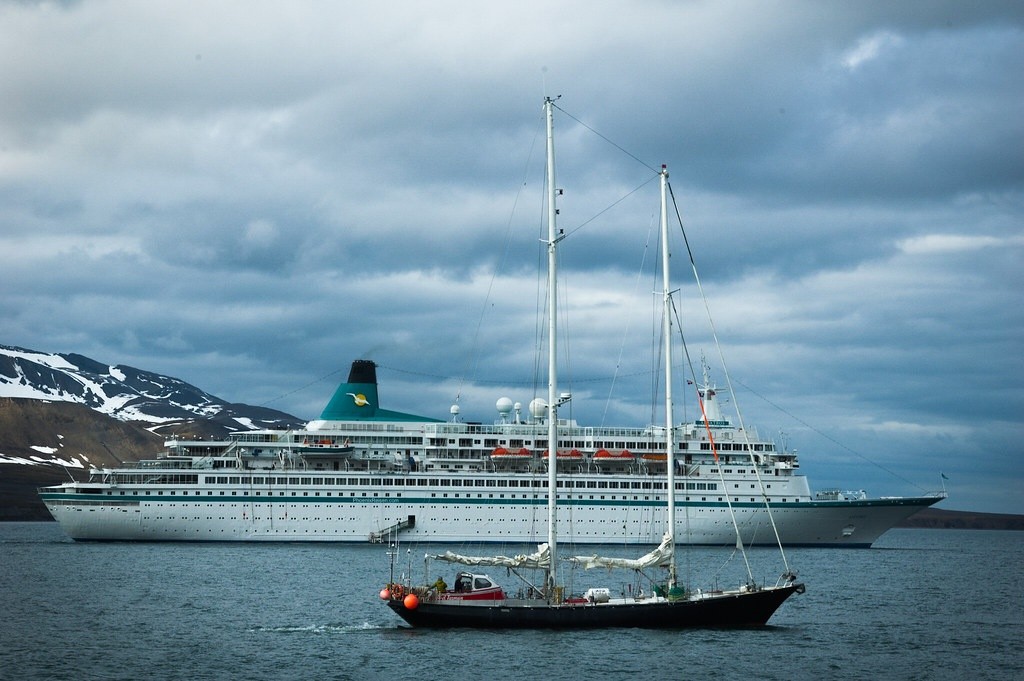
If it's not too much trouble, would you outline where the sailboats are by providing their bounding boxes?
[375,95,806,631]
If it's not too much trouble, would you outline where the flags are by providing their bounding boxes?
[942,473,949,479]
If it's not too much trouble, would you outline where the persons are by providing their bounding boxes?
[428,576,447,593]
[455,575,464,592]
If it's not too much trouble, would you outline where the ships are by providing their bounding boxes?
[37,347,948,548]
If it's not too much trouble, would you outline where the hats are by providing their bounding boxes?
[438,577,442,581]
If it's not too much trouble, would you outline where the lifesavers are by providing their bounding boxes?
[392,583,403,598]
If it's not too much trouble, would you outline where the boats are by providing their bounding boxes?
[642,452,669,464]
[592,447,635,461]
[491,447,532,459]
[297,434,355,456]
[543,446,583,459]
